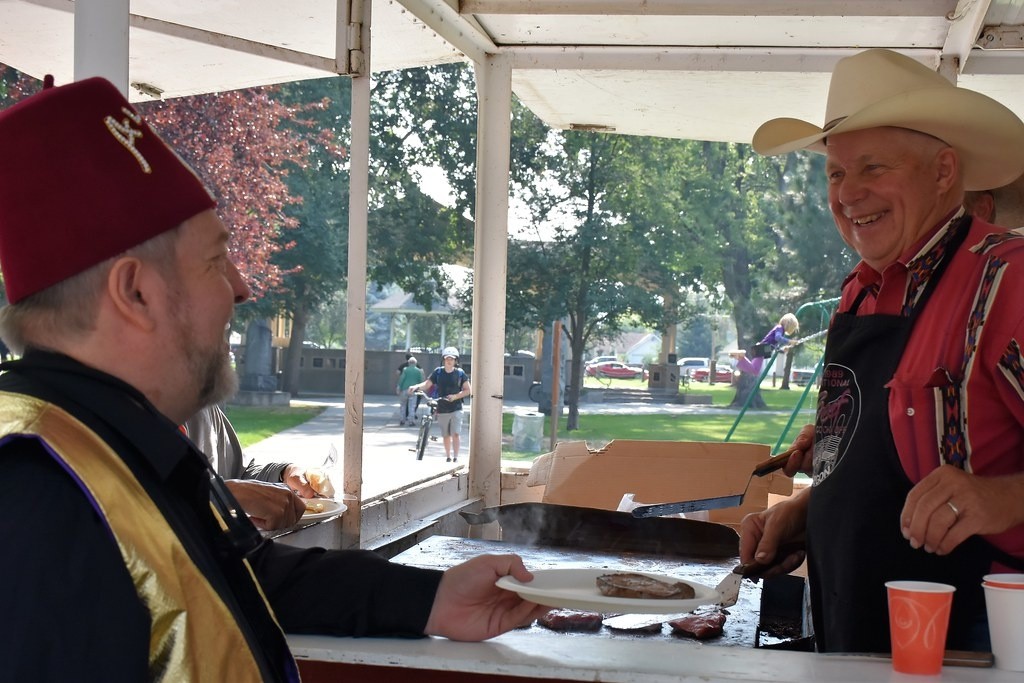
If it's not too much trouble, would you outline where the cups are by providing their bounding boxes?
[885,581,956,675]
[981,574,1024,672]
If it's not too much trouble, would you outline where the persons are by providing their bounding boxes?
[408,347,471,462]
[0,72,551,682]
[728,50,1024,655]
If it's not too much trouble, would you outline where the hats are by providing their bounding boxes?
[752,48,1024,192]
[982,173,1024,229]
[444,355,456,359]
[0,74,216,304]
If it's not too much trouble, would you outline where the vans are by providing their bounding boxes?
[677,356,710,379]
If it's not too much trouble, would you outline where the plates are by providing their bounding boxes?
[495,569,723,614]
[296,498,347,525]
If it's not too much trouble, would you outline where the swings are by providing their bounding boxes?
[751,329,829,359]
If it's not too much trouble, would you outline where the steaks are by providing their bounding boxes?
[668,610,728,639]
[596,574,695,599]
[638,624,663,635]
[536,609,604,631]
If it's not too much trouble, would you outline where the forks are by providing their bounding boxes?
[322,444,337,469]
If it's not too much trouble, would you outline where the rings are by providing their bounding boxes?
[946,502,961,517]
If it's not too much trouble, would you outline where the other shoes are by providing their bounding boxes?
[729,350,746,357]
[410,422,415,426]
[446,458,451,462]
[453,458,457,462]
[400,421,405,425]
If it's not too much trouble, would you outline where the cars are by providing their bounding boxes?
[585,356,649,382]
[694,366,736,383]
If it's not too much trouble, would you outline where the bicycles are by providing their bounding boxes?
[408,388,451,461]
[528,380,572,406]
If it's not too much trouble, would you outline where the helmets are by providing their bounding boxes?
[441,347,459,358]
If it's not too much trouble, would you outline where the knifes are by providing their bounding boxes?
[329,494,358,500]
[823,650,994,667]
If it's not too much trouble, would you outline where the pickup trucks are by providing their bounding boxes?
[790,363,824,388]
